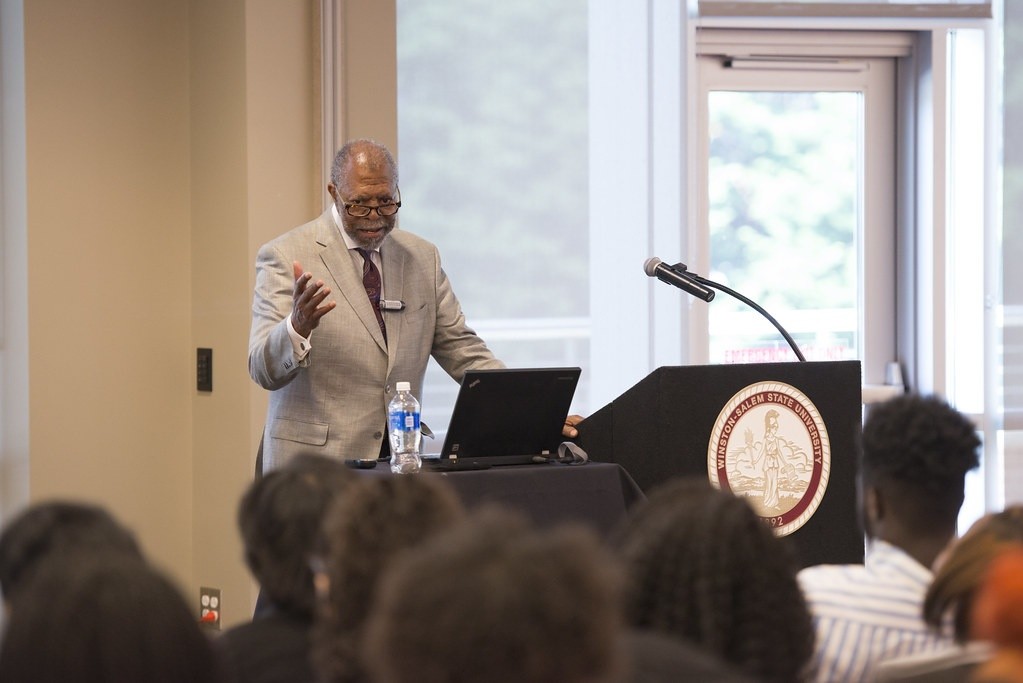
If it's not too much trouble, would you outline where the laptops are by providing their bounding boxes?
[377,366,582,471]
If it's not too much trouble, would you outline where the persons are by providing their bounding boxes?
[362,506,624,683]
[209,451,359,682]
[315,470,462,682]
[246,140,585,475]
[0,503,139,607]
[1,550,208,683]
[795,394,984,683]
[606,483,813,683]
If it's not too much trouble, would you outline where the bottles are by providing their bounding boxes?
[387,382,422,472]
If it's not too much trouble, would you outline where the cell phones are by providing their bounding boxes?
[344,459,377,469]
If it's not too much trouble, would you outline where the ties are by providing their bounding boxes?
[354,248,392,346]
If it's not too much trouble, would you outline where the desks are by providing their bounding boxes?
[345,460,646,532]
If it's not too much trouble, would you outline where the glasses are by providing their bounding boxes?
[328,181,402,218]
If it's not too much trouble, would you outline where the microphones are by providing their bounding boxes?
[643,257,715,303]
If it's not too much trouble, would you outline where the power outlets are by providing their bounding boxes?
[200,586,222,630]
